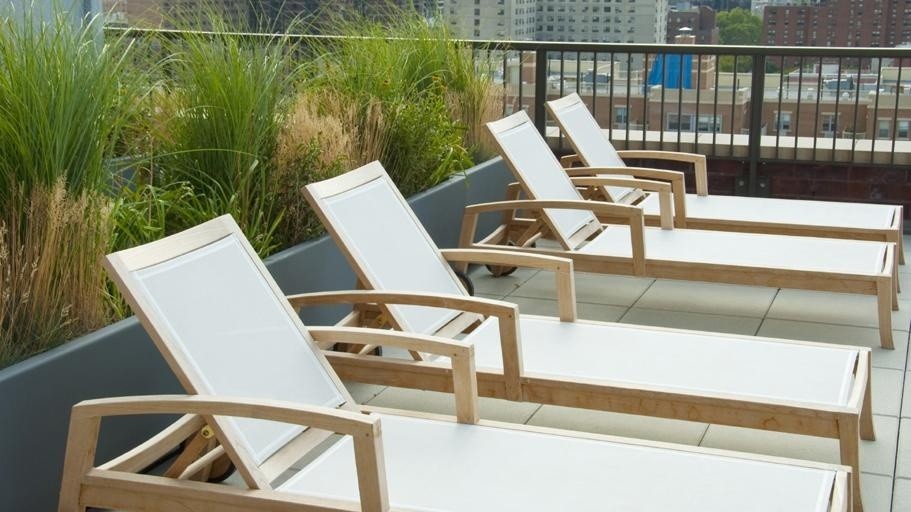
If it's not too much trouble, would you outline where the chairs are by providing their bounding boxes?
[544,91,905,292]
[455,108,899,350]
[57,211,855,512]
[300,160,877,512]
